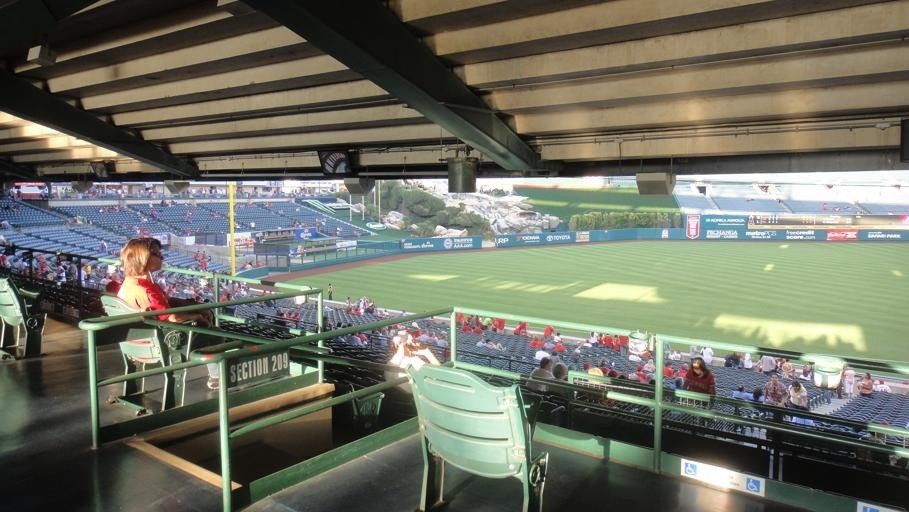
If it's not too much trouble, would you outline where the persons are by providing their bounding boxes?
[453,310,892,428]
[1,185,445,392]
[821,201,864,215]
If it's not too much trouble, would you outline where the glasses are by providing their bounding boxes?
[151,251,165,261]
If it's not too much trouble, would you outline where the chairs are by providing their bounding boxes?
[0,277,48,360]
[403,360,551,511]
[222,281,909,446]
[99,293,219,415]
